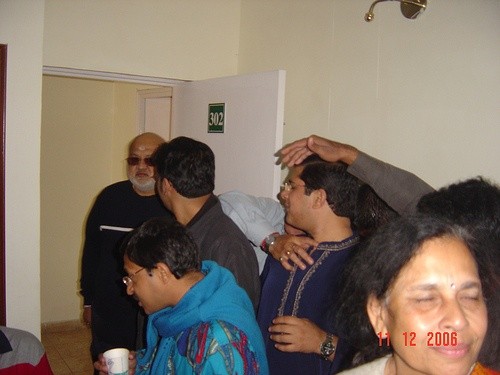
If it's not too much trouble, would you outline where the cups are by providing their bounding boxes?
[102,348,129,375]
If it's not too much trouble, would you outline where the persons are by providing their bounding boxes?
[256,154,392,375]
[274,135,500,372]
[93,215,268,375]
[76,133,177,375]
[219,172,317,271]
[333,212,500,375]
[157,136,262,322]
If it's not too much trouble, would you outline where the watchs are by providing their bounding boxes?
[264,232,276,247]
[319,331,335,362]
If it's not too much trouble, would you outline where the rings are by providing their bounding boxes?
[278,256,283,262]
[286,250,289,255]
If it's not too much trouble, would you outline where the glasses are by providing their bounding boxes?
[282,182,298,193]
[126,157,156,166]
[121,266,144,285]
